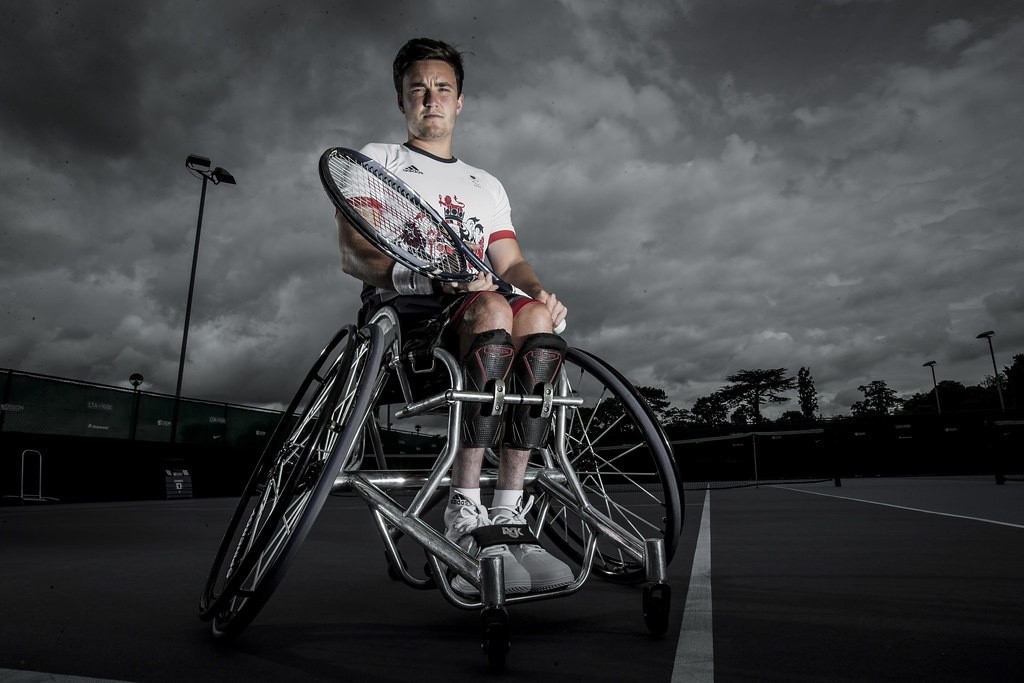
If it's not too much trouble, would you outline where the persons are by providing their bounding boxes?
[334,38,577,596]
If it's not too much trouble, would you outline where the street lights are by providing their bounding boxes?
[129,371,144,441]
[976,327,1008,411]
[922,358,945,415]
[169,148,240,455]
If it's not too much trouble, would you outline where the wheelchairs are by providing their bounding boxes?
[195,287,688,653]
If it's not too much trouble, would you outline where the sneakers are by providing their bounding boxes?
[444,489,576,596]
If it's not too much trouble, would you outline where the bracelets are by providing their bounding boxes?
[392,261,434,295]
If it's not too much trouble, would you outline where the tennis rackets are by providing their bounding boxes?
[317,146,570,336]
